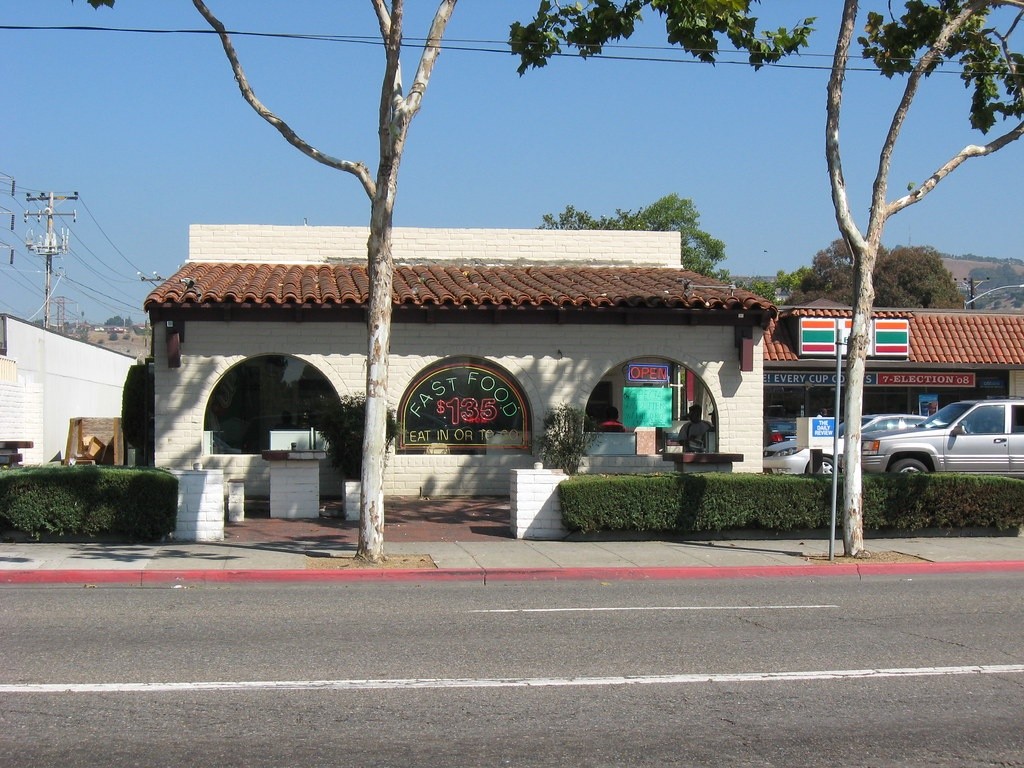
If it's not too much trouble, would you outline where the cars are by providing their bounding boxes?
[410,413,452,431]
[763,415,946,474]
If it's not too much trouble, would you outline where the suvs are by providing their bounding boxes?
[861,399,1023,474]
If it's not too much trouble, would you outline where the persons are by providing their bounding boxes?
[701,409,716,449]
[677,404,710,452]
[595,407,625,432]
